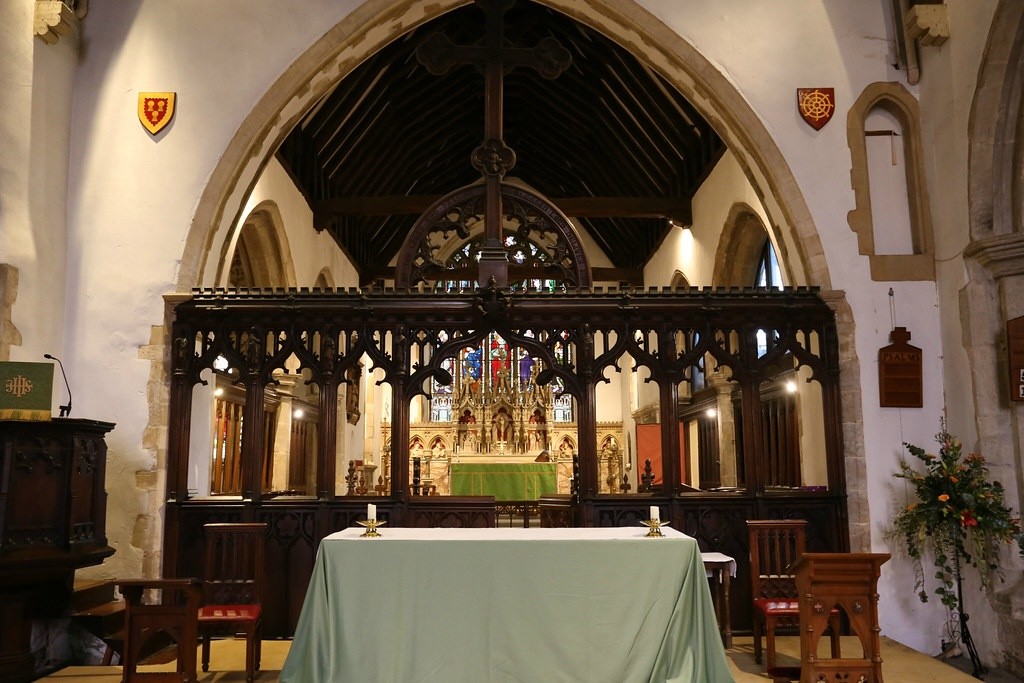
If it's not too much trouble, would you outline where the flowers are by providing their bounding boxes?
[886,415,1024,613]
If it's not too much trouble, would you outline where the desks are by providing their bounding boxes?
[699,551,733,652]
[278,525,736,683]
[450,461,558,501]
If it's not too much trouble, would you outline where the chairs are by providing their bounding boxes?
[191,522,269,682]
[746,519,841,678]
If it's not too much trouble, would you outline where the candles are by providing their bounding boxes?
[367,502,376,520]
[457,446,460,454]
[549,442,552,452]
[650,505,660,522]
